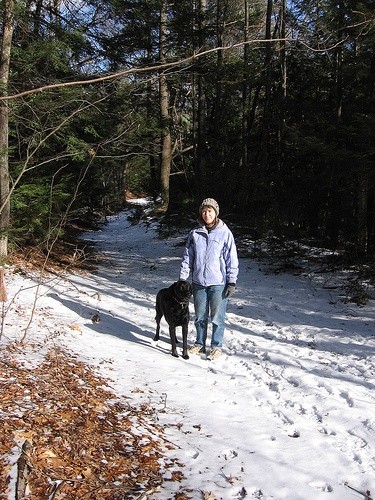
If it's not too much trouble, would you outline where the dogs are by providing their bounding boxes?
[153,278,194,360]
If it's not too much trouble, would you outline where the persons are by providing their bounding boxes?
[179,198,239,359]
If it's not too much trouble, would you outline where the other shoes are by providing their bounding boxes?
[207,348,222,359]
[188,345,205,353]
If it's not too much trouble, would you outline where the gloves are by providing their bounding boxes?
[225,284,235,298]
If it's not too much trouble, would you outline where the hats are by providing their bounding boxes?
[199,198,219,216]
